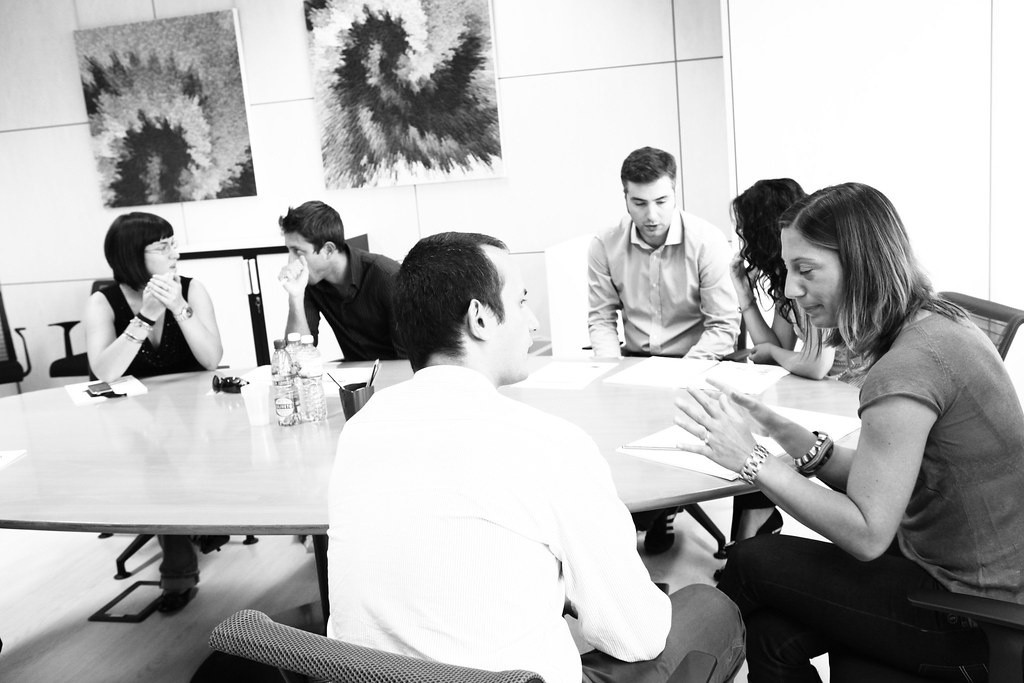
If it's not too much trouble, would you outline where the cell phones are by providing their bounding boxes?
[88,382,113,396]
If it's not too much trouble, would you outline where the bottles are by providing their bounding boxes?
[270,338,298,426]
[297,335,328,421]
[285,332,301,420]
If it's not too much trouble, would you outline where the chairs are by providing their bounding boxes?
[49,280,117,378]
[209,610,718,683]
[0,286,31,395]
[936,291,1024,363]
[829,591,1024,683]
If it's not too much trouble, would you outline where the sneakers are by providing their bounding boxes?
[644,506,679,552]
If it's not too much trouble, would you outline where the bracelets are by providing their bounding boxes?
[741,443,770,487]
[739,296,757,314]
[125,312,155,344]
[793,430,834,479]
[703,430,712,445]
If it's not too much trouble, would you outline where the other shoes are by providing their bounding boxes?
[156,588,194,614]
[722,509,782,551]
[198,534,230,554]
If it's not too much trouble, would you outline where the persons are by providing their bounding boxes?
[729,177,875,390]
[588,146,742,361]
[673,180,1024,683]
[84,212,231,613]
[324,230,746,683]
[279,200,417,362]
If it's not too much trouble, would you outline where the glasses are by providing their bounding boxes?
[144,241,178,255]
[212,375,248,392]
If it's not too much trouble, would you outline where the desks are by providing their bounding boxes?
[0,354,863,634]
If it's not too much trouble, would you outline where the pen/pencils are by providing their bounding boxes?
[621,444,682,451]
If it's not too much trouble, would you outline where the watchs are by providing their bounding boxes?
[173,304,193,322]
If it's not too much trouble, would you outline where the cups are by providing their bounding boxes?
[339,382,374,422]
